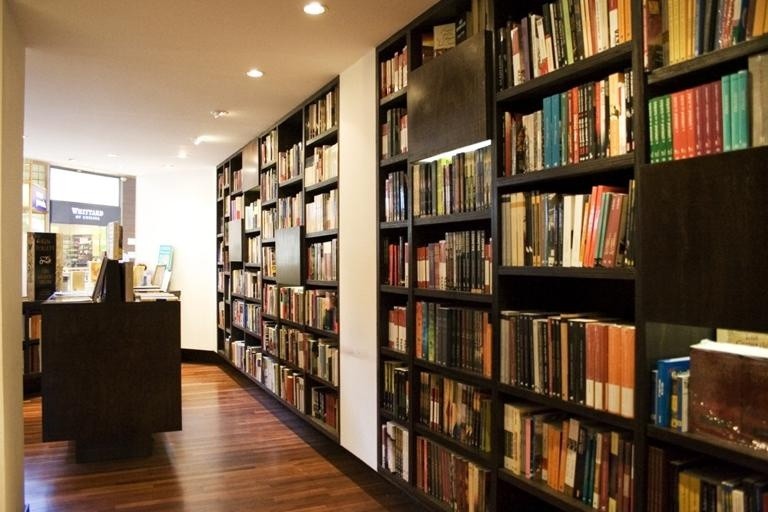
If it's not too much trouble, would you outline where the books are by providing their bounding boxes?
[215,83,340,443]
[91,221,134,304]
[378,0,768,511]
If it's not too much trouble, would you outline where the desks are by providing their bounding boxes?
[41,261,182,444]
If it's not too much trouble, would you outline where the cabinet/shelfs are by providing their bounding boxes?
[217,74,340,444]
[376,0,767,510]
[22,301,43,400]
